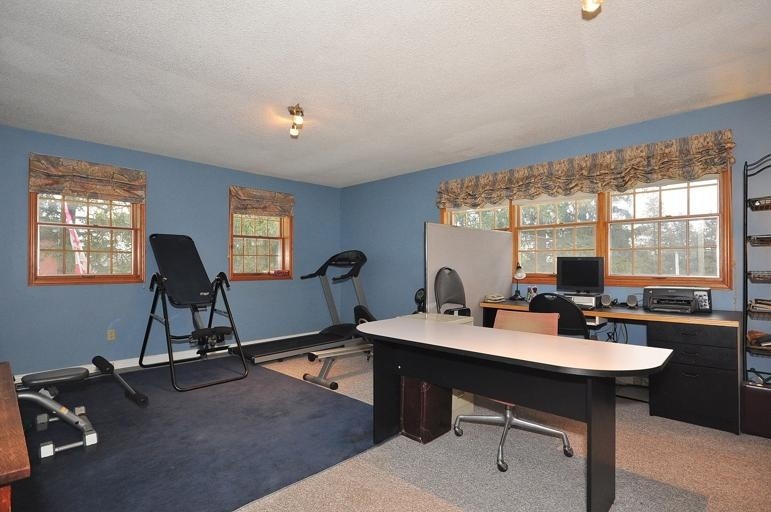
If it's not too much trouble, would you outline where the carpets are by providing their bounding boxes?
[0,349,416,512]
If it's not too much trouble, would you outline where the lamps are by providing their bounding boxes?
[580,0,604,22]
[277,102,310,143]
[514,263,526,302]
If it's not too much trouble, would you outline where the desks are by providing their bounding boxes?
[356,314,674,512]
[476,295,742,435]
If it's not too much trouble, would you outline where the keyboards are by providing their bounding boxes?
[576,304,594,311]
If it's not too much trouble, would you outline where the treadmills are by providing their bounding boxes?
[228,250,378,364]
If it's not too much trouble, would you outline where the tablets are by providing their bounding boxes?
[556,256,605,293]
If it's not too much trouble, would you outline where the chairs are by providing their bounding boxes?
[525,295,592,340]
[454,307,578,473]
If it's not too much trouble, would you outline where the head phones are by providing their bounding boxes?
[600,295,638,308]
